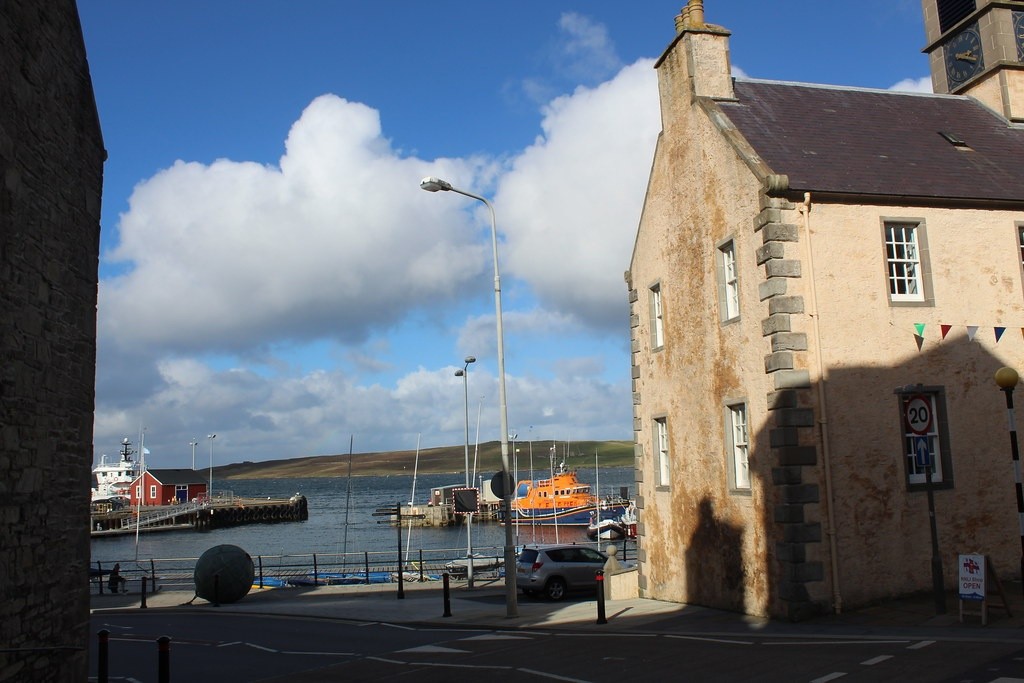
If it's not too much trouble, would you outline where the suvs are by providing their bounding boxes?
[514,544,633,601]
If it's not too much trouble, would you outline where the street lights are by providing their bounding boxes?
[509,433,520,549]
[188,436,198,471]
[454,356,477,589]
[207,433,217,505]
[418,175,520,617]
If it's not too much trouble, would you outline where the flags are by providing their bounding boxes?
[143,446,150,454]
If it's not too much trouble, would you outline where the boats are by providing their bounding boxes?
[500,431,637,540]
[91,436,141,501]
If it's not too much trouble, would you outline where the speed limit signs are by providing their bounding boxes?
[906,396,933,434]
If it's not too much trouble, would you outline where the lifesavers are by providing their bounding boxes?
[207,498,308,527]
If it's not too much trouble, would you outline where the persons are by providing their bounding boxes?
[108,564,128,593]
